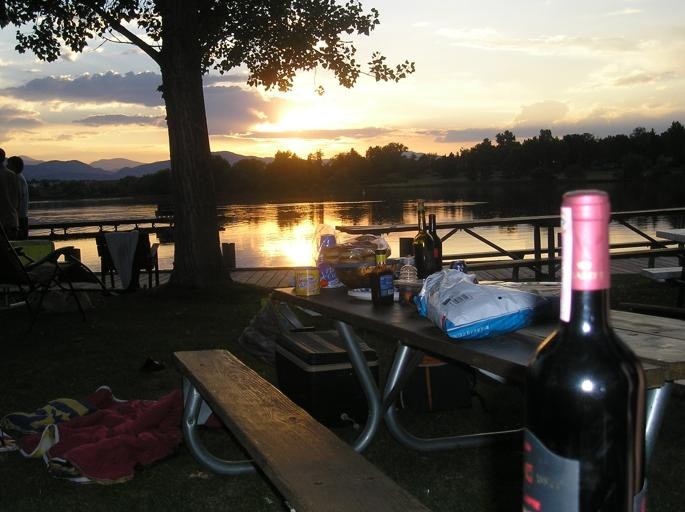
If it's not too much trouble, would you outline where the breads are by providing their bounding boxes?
[324,235,390,264]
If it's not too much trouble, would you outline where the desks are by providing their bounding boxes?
[335,206,683,282]
[273,279,684,509]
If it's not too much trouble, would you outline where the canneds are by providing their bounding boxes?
[451,260,467,274]
[295,268,320,296]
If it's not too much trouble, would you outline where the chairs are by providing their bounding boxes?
[0,221,160,335]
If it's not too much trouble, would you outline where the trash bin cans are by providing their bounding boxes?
[222,242,235,268]
[399,238,415,256]
[66,248,81,262]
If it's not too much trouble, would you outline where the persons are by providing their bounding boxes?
[0,149,28,240]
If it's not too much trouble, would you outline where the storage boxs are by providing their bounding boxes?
[268,329,380,426]
[409,352,474,412]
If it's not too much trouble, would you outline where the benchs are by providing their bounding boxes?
[336,237,684,280]
[173,346,430,510]
[442,239,685,309]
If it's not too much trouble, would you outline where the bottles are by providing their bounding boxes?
[370,249,395,308]
[428,213,442,272]
[412,197,434,278]
[518,189,650,512]
